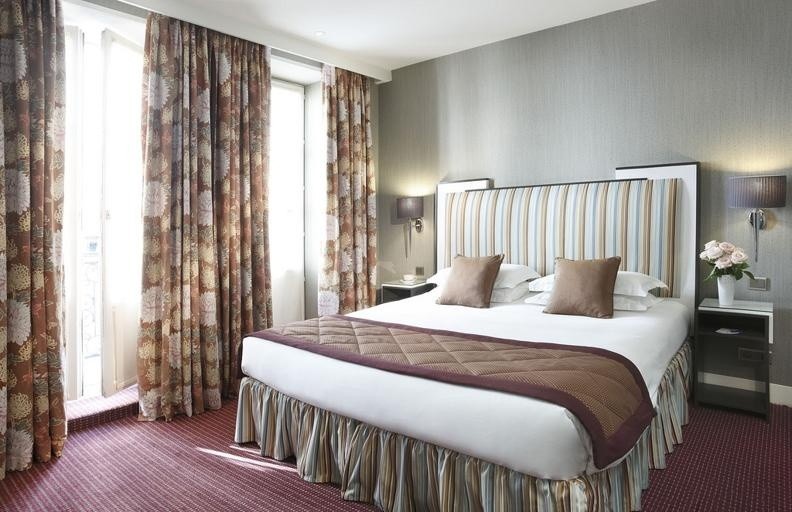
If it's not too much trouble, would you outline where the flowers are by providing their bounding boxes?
[698,239,755,281]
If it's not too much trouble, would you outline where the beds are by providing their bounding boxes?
[240,162,702,512]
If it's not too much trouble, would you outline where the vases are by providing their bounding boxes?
[716,274,737,306]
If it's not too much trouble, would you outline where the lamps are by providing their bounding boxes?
[726,173,789,230]
[396,196,425,233]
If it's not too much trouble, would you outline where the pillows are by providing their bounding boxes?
[425,253,667,321]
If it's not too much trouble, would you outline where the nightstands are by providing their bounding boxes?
[380,278,435,305]
[693,296,775,425]
